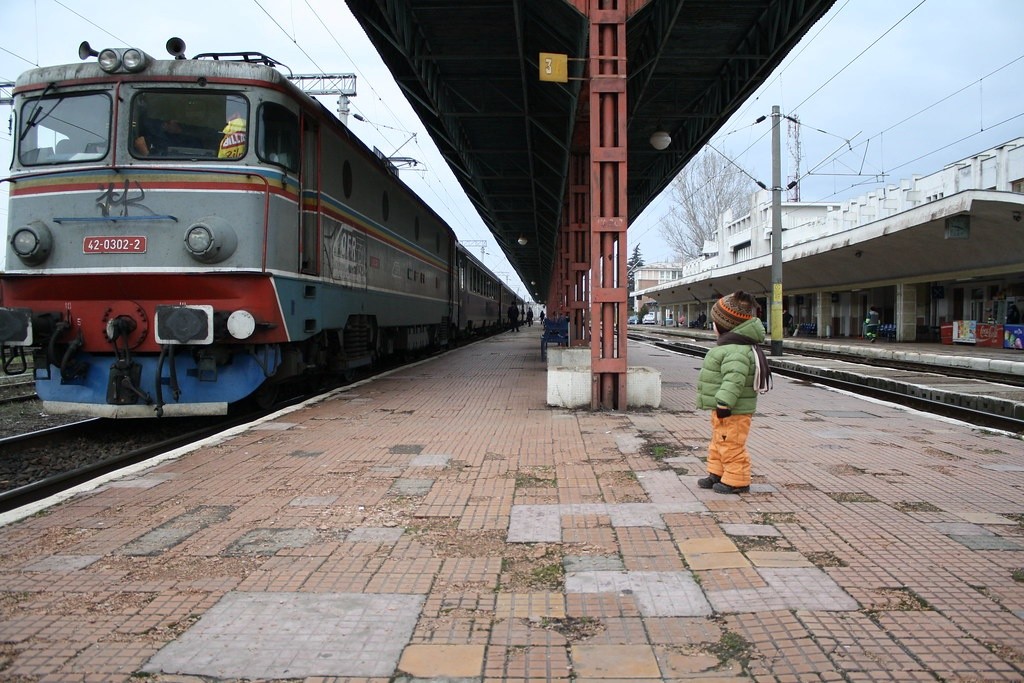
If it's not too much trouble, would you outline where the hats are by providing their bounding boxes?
[710,291,762,331]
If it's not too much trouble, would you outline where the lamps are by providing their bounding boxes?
[1013,211,1021,222]
[517,233,528,245]
[855,250,864,257]
[531,280,541,303]
[649,124,671,151]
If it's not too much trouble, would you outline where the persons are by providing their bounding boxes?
[696,311,707,330]
[865,306,881,342]
[696,287,774,495]
[782,309,794,338]
[134,99,203,156]
[540,311,544,323]
[527,309,533,326]
[507,301,519,332]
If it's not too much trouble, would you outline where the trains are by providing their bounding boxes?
[0,35,537,422]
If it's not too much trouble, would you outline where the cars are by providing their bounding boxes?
[627,315,639,324]
[642,314,656,325]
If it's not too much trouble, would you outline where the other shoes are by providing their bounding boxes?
[713,481,750,494]
[871,338,875,343]
[697,474,721,488]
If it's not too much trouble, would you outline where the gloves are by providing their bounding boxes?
[715,402,731,418]
